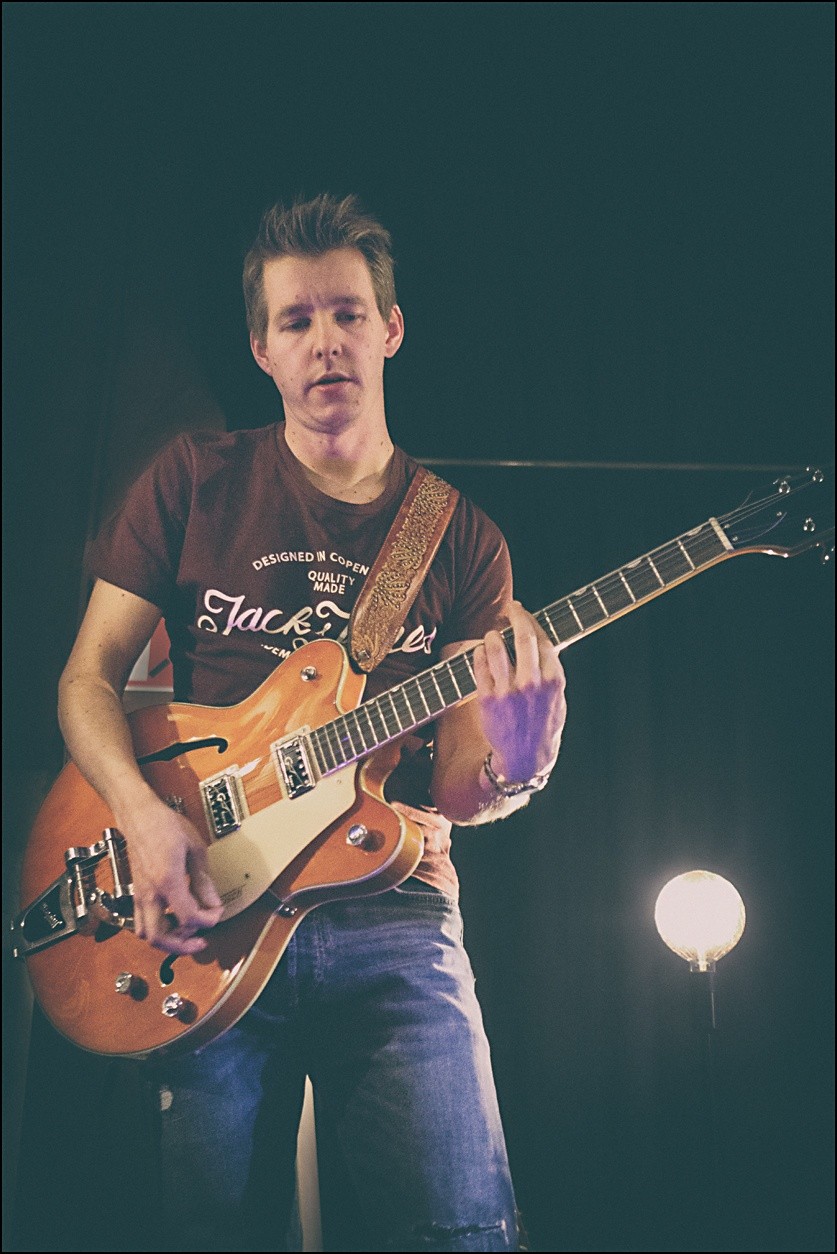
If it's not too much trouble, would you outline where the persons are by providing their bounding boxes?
[55,194,571,1254]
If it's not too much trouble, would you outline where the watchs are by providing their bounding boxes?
[483,748,553,797]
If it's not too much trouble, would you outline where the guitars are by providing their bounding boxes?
[9,460,837,1065]
